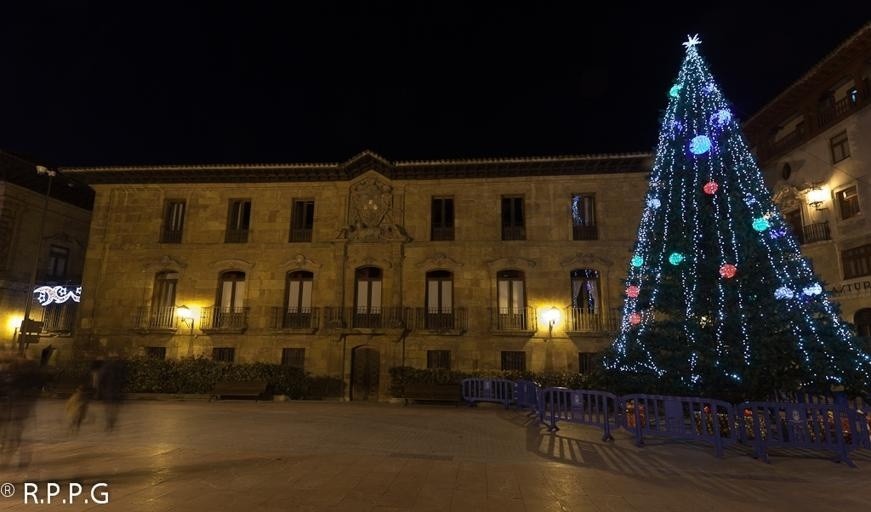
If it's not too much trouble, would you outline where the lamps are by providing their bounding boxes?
[177,305,194,330]
[805,184,829,211]
[545,306,560,336]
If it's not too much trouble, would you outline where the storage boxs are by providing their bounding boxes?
[615,407,645,428]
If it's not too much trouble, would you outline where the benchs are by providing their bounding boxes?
[42,368,113,401]
[208,382,272,405]
[400,382,463,409]
